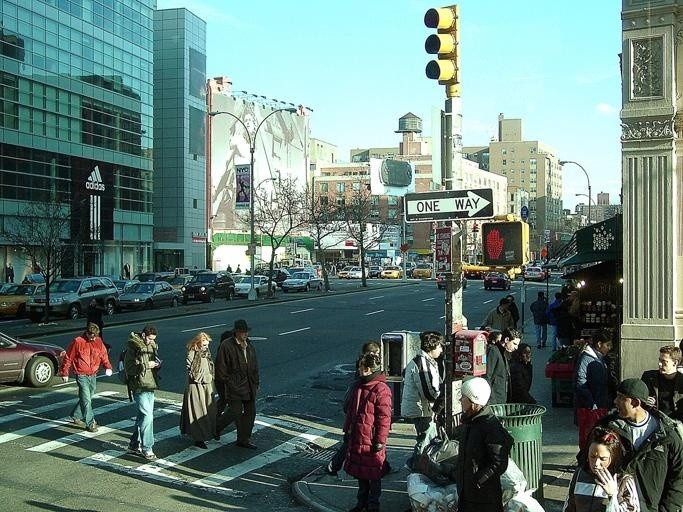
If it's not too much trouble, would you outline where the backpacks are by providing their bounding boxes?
[117,349,128,384]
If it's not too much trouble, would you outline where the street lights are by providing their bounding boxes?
[574,192,599,223]
[530,211,557,239]
[210,108,299,301]
[559,160,593,226]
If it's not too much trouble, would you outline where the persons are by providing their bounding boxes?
[124,262,130,280]
[34,261,40,273]
[227,110,256,165]
[180,319,260,449]
[227,264,241,273]
[61,321,113,432]
[531,283,617,450]
[124,326,162,460]
[562,342,683,512]
[325,296,534,510]
[8,262,14,282]
[85,299,111,355]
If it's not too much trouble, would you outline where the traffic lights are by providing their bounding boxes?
[483,222,528,266]
[424,5,461,82]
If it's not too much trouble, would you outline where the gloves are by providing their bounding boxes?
[372,442,383,451]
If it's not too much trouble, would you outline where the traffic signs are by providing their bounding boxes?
[405,190,493,222]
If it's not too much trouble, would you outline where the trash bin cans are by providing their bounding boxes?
[485,402,547,502]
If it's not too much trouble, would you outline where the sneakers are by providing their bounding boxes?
[195,441,207,449]
[142,450,156,460]
[350,502,365,512]
[71,415,81,424]
[128,448,143,454]
[214,431,219,440]
[87,427,98,432]
[389,467,400,474]
[236,441,256,449]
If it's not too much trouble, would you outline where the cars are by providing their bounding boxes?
[337,263,434,279]
[524,258,561,281]
[0,330,70,389]
[483,272,512,291]
[436,273,468,289]
[463,262,486,279]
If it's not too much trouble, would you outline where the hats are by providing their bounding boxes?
[617,379,654,408]
[462,377,490,406]
[232,320,251,334]
